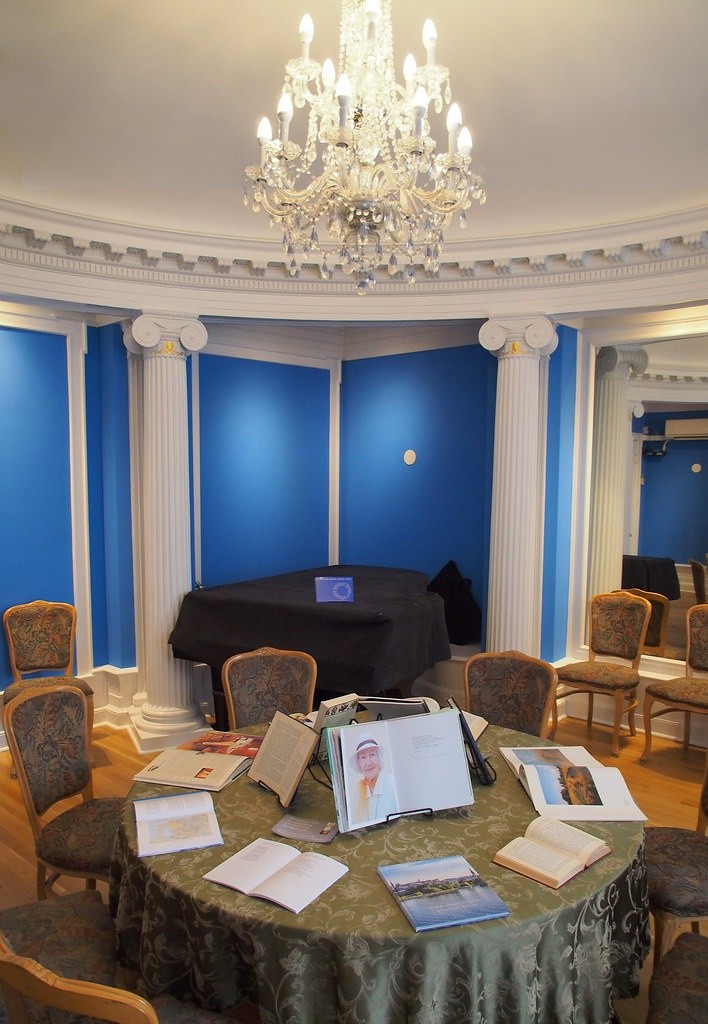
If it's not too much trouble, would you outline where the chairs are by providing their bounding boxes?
[690,558,708,606]
[643,767,708,1023]
[464,649,558,740]
[641,603,708,762]
[3,684,128,902]
[222,647,316,728]
[0,600,94,780]
[0,890,238,1024]
[549,591,652,757]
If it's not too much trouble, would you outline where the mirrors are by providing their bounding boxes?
[577,316,708,678]
[611,588,669,657]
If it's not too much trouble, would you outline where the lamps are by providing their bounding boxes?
[243,1,488,296]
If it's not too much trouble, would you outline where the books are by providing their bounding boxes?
[377,855,511,932]
[203,838,349,914]
[132,693,494,834]
[492,814,612,891]
[132,790,224,857]
[498,746,650,821]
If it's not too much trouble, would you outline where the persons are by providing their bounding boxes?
[350,733,398,821]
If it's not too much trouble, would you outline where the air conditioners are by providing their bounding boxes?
[664,419,708,441]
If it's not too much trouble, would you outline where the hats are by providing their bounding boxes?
[348,736,384,774]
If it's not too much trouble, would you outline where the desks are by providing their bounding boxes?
[107,723,651,1024]
